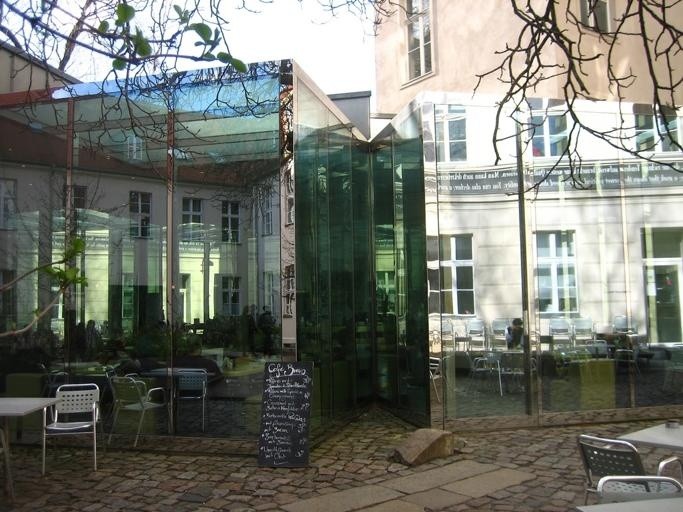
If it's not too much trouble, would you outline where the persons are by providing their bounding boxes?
[172,333,224,397]
[236,302,276,361]
[60,308,104,364]
[505,317,525,351]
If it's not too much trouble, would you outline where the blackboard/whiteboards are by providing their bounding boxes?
[257,361,313,469]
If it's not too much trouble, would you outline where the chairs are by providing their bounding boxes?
[429,316,683,410]
[42,383,107,476]
[576,433,683,506]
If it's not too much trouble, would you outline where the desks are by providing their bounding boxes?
[615,424,683,452]
[576,497,683,512]
[0,397,63,497]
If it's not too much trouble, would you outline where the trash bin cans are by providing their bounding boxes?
[614,349,635,409]
[635,346,670,407]
